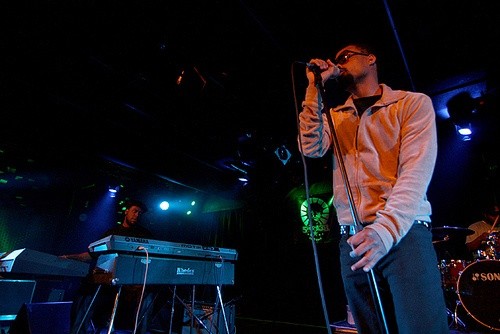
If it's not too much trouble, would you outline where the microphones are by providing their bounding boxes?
[302,61,329,74]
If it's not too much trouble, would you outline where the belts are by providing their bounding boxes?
[340,221,430,235]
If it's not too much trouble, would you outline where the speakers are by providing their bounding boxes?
[8,301,96,334]
[0,279,37,320]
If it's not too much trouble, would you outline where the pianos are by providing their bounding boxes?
[87,235,238,262]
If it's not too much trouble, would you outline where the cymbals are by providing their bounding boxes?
[432,226,474,236]
[432,238,459,247]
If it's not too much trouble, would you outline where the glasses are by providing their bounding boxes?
[335,50,368,64]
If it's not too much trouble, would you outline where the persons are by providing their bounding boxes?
[297,43,450,334]
[59,198,146,334]
[463,198,500,265]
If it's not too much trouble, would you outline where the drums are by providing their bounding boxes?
[441,257,472,287]
[455,257,500,333]
